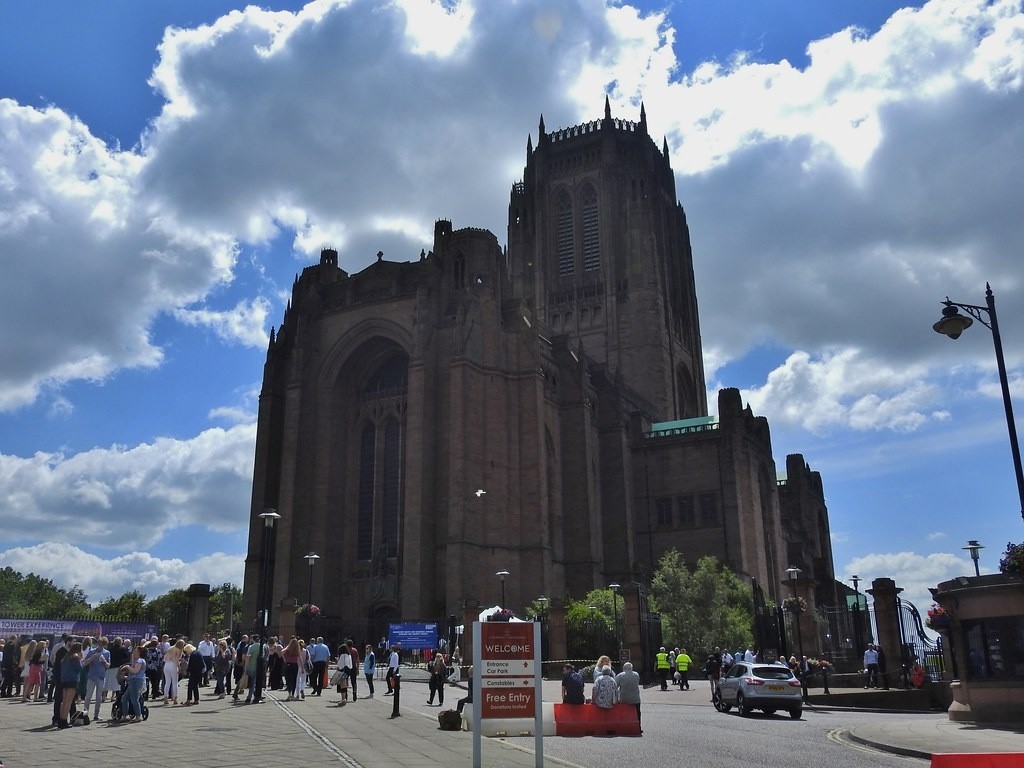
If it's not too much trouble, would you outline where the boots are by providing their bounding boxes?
[61,720,72,729]
[51,717,59,727]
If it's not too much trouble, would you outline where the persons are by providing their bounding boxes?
[714,645,757,677]
[707,657,719,702]
[911,666,924,688]
[337,640,360,705]
[385,643,402,695]
[263,635,331,700]
[426,653,447,706]
[457,668,473,713]
[614,662,643,733]
[365,646,375,698]
[0,633,147,728]
[863,644,889,690]
[141,629,265,705]
[654,647,694,690]
[780,656,813,687]
[592,656,618,708]
[562,664,585,704]
[447,652,461,681]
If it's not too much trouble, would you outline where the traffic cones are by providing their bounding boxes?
[322,667,332,689]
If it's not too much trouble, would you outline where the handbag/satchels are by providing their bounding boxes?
[330,671,348,689]
[304,660,314,674]
[438,709,462,730]
[239,673,249,689]
[70,711,90,725]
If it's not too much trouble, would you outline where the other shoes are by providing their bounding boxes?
[115,719,128,724]
[439,704,442,706]
[384,690,393,694]
[427,701,432,705]
[0,681,211,719]
[130,717,142,723]
[214,682,374,707]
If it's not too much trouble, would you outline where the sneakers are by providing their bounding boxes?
[94,716,103,721]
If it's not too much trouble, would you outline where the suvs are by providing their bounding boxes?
[712,661,803,719]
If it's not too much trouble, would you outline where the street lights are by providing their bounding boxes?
[258,508,281,638]
[961,540,986,578]
[933,282,1024,518]
[495,570,510,610]
[785,564,808,697]
[608,581,621,673]
[538,596,548,626]
[304,552,320,640]
[849,575,862,659]
[588,605,597,657]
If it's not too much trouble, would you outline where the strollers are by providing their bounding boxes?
[111,676,149,720]
